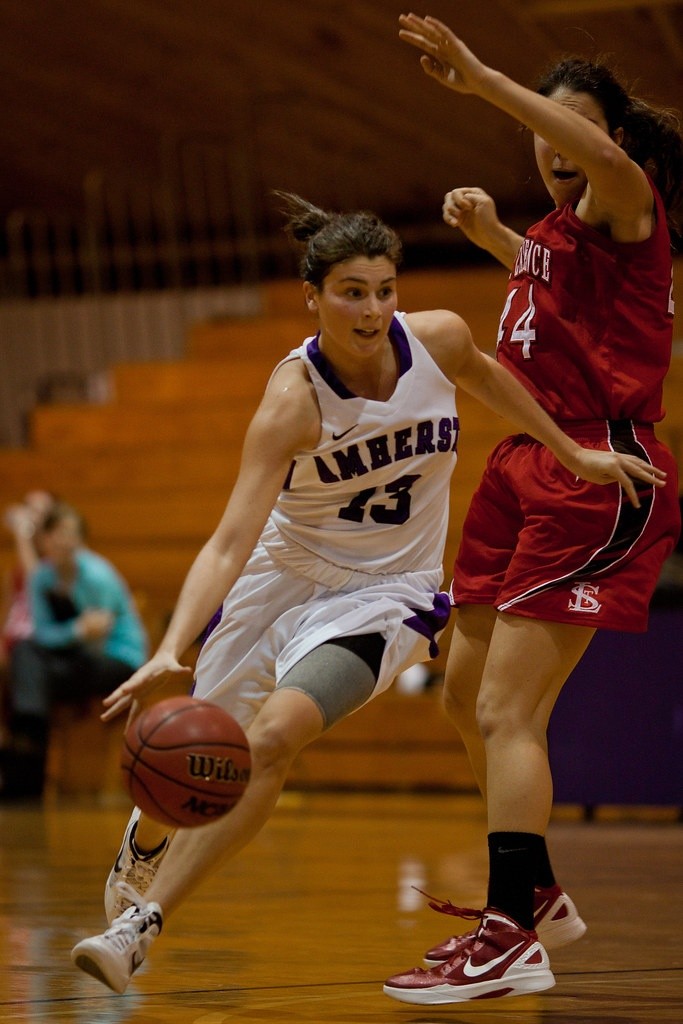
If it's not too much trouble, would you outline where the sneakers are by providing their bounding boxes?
[423,884,589,969]
[70,898,166,997]
[104,804,171,929]
[382,906,556,1007]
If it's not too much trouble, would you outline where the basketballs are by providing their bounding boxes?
[121,695,251,828]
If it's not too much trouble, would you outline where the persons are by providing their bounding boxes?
[70,188,668,993]
[2,488,54,641]
[382,10,677,1005]
[0,503,150,807]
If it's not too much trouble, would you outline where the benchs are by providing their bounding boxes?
[0,254,682,803]
